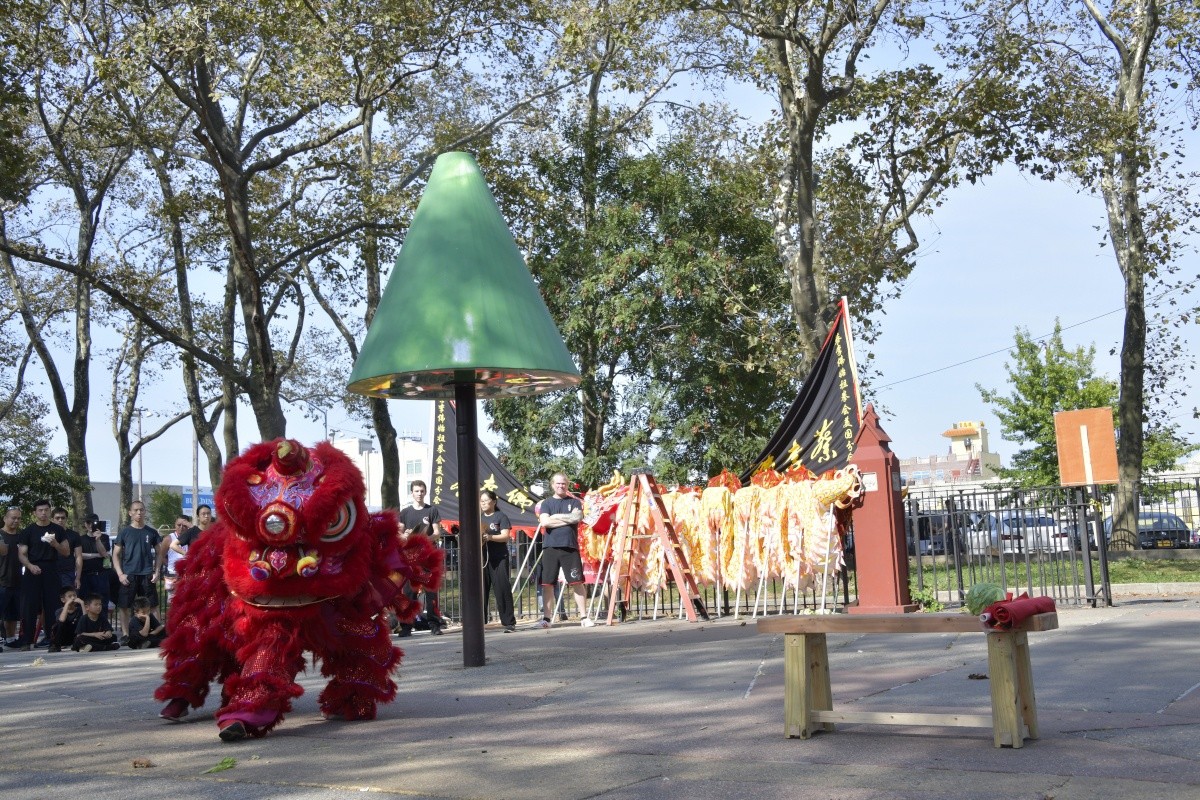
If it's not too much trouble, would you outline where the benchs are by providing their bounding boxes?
[757,611,1060,748]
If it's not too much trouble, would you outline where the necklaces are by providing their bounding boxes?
[174,532,179,540]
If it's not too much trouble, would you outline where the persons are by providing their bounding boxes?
[0,500,216,653]
[398,480,443,637]
[479,490,516,633]
[533,472,595,629]
[908,497,970,557]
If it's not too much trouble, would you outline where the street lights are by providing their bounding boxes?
[137,407,153,503]
[328,429,335,447]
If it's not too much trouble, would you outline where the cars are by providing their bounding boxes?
[967,510,1070,554]
[1063,512,1190,550]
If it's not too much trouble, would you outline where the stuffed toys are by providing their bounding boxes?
[154,434,447,742]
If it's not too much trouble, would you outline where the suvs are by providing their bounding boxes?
[903,515,970,555]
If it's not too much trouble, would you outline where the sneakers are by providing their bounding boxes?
[504,624,516,633]
[219,719,246,741]
[159,701,190,721]
[531,619,553,629]
[582,616,594,627]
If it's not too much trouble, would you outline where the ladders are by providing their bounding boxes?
[606,474,711,626]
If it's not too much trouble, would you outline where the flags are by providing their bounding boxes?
[430,395,546,543]
[740,295,863,490]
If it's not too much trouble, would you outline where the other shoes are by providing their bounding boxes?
[4,640,26,648]
[79,645,92,652]
[103,641,120,651]
[321,711,344,719]
[49,645,61,652]
[136,640,151,649]
[120,636,130,646]
[431,624,442,635]
[399,628,412,636]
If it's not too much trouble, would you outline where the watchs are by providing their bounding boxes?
[155,567,161,573]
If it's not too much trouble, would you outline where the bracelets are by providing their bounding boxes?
[489,534,493,541]
[118,574,124,578]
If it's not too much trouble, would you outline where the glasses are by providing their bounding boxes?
[481,498,492,503]
[55,516,66,520]
[6,505,23,513]
[86,521,90,524]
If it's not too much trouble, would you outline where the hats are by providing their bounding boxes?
[85,514,99,521]
